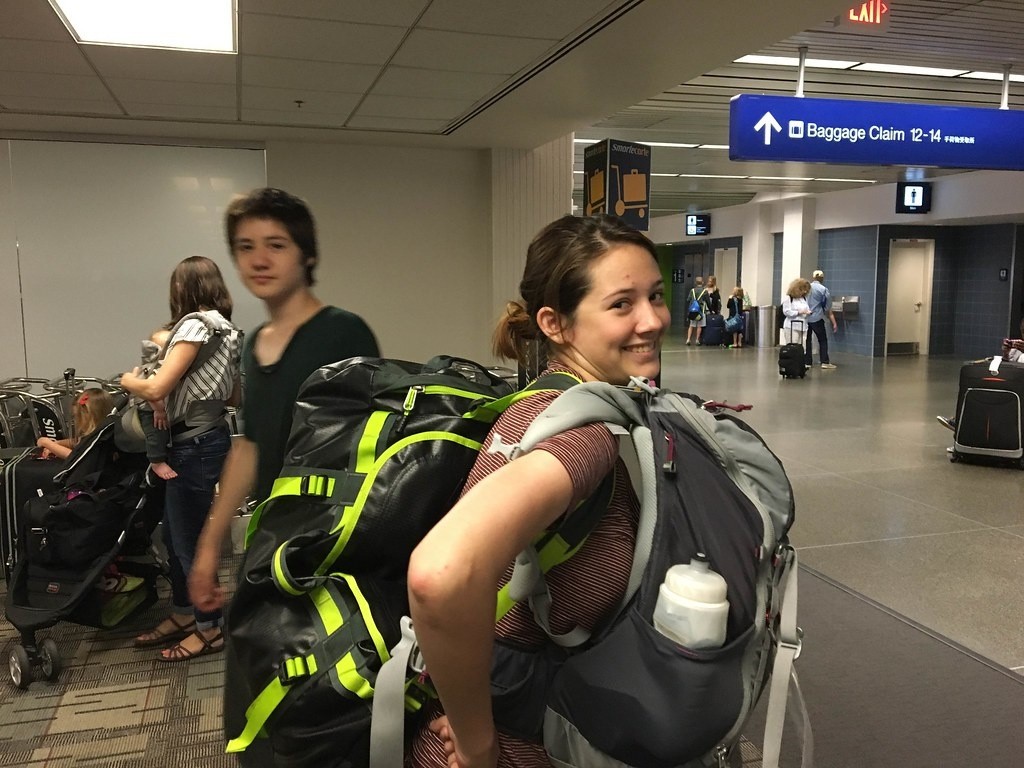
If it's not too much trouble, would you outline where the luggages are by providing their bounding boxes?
[701,309,726,346]
[953,355,1024,470]
[779,319,806,380]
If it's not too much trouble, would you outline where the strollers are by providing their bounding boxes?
[8,413,166,690]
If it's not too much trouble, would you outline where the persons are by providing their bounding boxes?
[937,320,1024,453]
[782,269,838,368]
[686,275,744,348]
[37,256,245,662]
[188,188,380,768]
[404,212,673,768]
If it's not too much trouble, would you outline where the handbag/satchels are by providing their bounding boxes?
[42,483,128,563]
[725,314,742,333]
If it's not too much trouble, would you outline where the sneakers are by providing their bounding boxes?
[821,363,838,369]
[804,364,812,369]
[937,415,956,431]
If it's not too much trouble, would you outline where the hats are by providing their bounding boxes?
[813,270,824,278]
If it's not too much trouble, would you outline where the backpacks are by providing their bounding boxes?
[776,294,806,328]
[524,378,815,768]
[224,356,617,768]
[688,288,706,322]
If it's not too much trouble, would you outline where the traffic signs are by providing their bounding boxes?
[729,93,1024,170]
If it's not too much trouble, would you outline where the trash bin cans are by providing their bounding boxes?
[757,305,776,348]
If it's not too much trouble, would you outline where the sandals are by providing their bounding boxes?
[132,614,199,647]
[157,630,226,663]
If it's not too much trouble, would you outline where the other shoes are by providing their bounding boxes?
[728,344,742,348]
[946,447,955,453]
[685,341,701,346]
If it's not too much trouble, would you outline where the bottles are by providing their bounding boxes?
[652,552,730,650]
[719,343,727,350]
[841,297,845,303]
